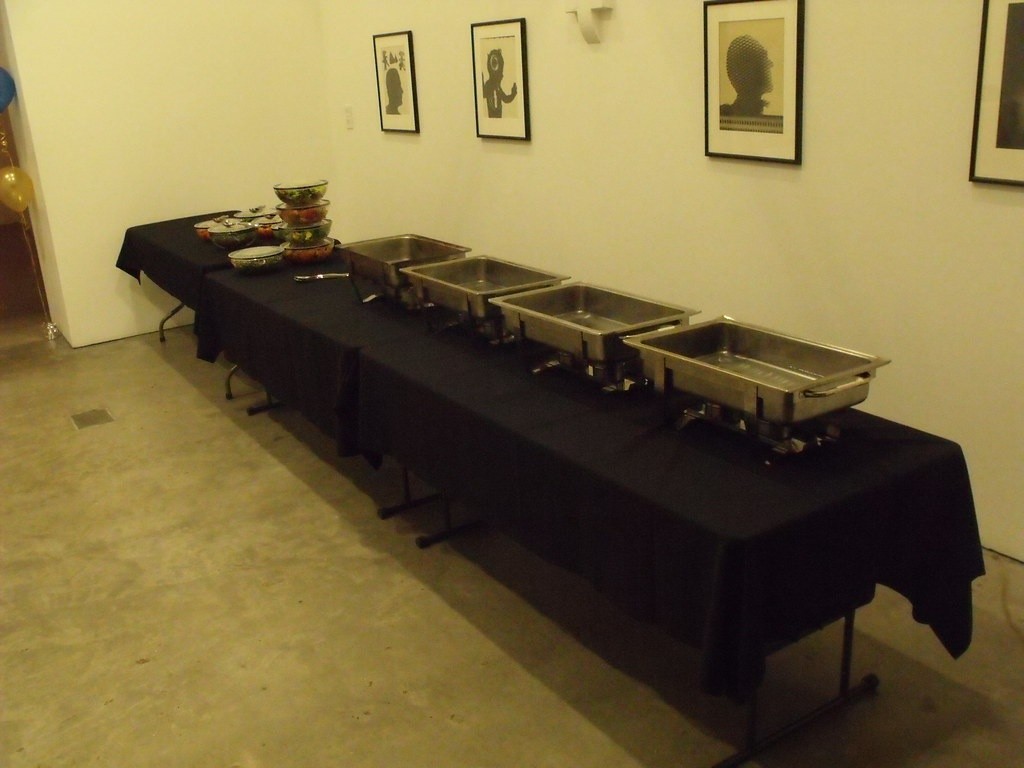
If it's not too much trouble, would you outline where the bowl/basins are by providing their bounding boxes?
[277,200,329,225]
[194,215,243,240]
[207,219,256,248]
[280,218,332,245]
[233,207,280,221]
[273,182,327,205]
[228,246,284,276]
[252,218,281,238]
[282,238,335,263]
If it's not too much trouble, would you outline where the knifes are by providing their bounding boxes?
[294,274,348,280]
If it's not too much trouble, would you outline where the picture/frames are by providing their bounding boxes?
[469,17,532,142]
[703,0,803,168]
[373,31,421,135]
[968,0,1024,187]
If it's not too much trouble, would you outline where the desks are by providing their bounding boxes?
[116,209,986,768]
